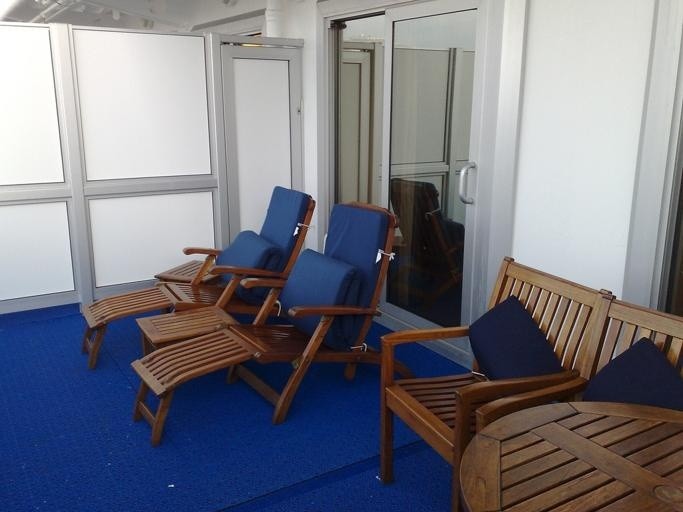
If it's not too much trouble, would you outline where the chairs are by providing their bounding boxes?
[473,292,683,435]
[389,177,464,302]
[378,254,614,510]
[131,199,415,446]
[79,187,320,375]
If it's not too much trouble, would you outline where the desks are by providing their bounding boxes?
[458,400,682,511]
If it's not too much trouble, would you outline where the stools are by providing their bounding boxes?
[136,305,239,361]
[152,258,221,284]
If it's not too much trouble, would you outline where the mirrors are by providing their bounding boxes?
[386,7,479,326]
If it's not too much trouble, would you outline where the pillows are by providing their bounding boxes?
[584,332,683,411]
[466,292,568,387]
